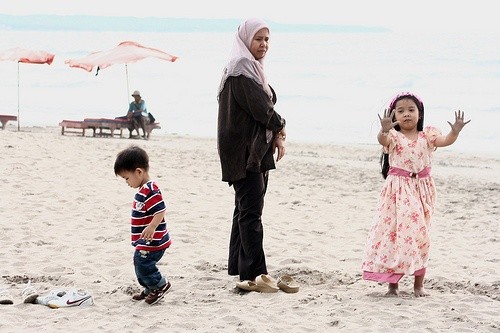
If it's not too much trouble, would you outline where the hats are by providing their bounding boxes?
[132,90,140,96]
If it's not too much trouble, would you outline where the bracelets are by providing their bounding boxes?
[275,134,286,141]
[379,127,390,136]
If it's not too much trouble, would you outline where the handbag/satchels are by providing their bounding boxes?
[148,112,155,123]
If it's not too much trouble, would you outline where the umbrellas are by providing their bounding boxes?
[0,45,54,132]
[63,39,180,138]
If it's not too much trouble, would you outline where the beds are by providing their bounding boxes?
[82,118,157,139]
[1,115,17,129]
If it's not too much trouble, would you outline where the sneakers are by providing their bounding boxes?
[47,289,94,309]
[20,280,40,304]
[131,289,149,302]
[0,289,14,304]
[36,287,66,305]
[145,277,172,306]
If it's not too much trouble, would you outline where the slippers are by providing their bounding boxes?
[276,274,300,293]
[236,274,280,293]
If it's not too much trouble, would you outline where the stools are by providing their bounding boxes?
[61,120,85,136]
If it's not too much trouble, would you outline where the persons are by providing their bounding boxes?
[113,144,172,306]
[215,15,287,295]
[122,89,151,140]
[361,91,472,298]
